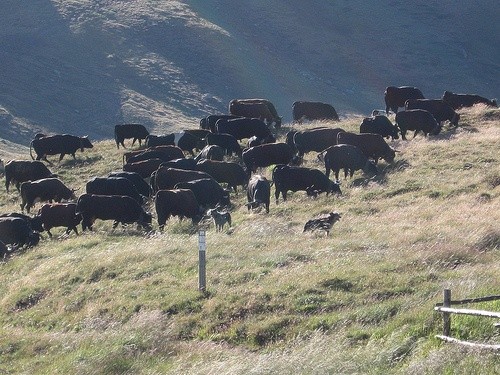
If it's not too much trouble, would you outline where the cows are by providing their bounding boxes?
[0,86,497,259]
